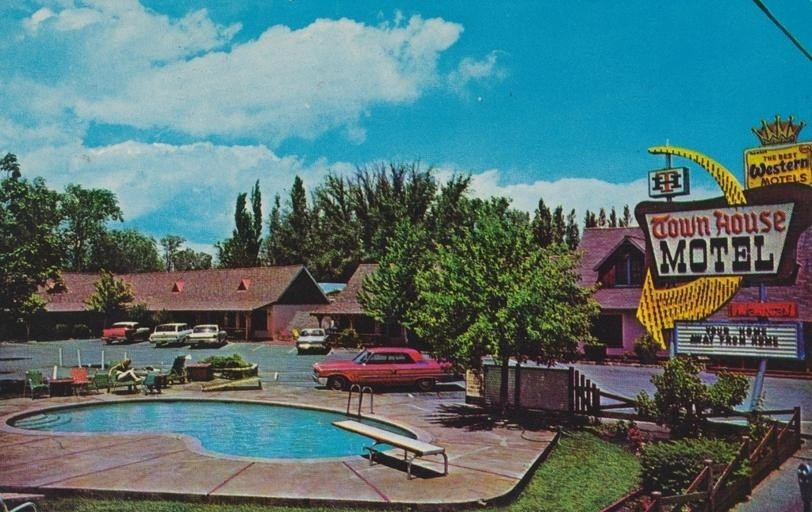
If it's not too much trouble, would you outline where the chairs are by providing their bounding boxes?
[23,356,186,400]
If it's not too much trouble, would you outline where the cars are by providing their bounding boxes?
[149,323,228,348]
[312,346,454,392]
[297,329,331,353]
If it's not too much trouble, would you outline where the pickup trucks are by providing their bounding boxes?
[103,322,151,345]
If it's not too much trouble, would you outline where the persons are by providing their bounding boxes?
[107,358,144,383]
[142,366,161,394]
